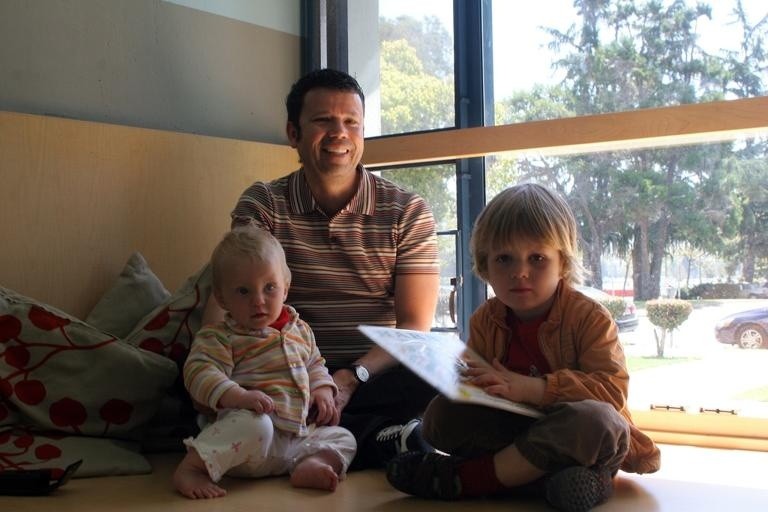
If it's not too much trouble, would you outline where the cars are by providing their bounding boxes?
[716,307,768,349]
[573,285,639,334]
[683,281,767,301]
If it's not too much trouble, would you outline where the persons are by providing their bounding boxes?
[382,181,663,511]
[170,223,361,500]
[186,66,439,473]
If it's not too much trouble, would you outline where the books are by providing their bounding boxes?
[355,320,549,421]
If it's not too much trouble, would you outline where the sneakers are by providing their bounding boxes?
[377,417,436,455]
[386,456,462,500]
[546,464,613,512]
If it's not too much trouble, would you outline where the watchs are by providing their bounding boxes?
[346,364,371,386]
